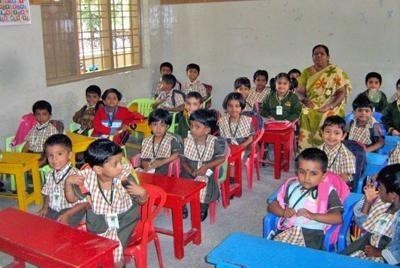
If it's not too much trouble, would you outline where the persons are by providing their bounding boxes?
[382,81,398,136]
[228,77,264,166]
[152,75,183,111]
[35,131,90,227]
[245,70,272,110]
[289,69,302,99]
[91,88,144,146]
[10,99,60,196]
[352,72,388,109]
[74,84,104,136]
[338,163,400,268]
[175,92,203,139]
[181,63,207,109]
[294,43,354,158]
[141,108,184,176]
[64,138,150,268]
[216,92,255,158]
[261,72,301,165]
[344,98,384,153]
[182,110,225,220]
[266,148,344,252]
[316,116,355,188]
[154,62,181,99]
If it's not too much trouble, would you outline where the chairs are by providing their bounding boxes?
[0,85,400,267]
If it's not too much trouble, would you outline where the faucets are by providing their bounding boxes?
[200,203,209,221]
[183,203,188,219]
